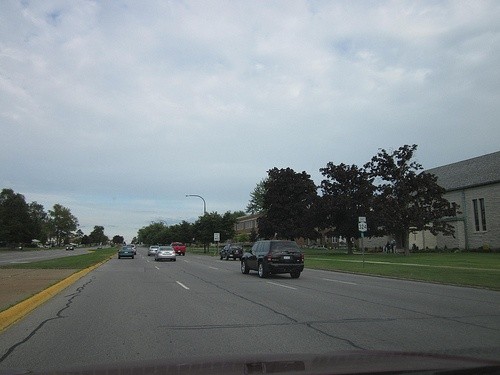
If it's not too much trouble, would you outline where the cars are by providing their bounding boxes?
[65,244,74,250]
[154,246,176,261]
[97,245,103,250]
[126,244,136,255]
[219,244,243,261]
[147,246,160,256]
[241,239,304,278]
[38,243,51,249]
[118,246,135,259]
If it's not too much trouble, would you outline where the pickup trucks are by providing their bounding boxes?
[170,242,186,255]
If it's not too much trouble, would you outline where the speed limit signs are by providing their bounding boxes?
[358,215,368,233]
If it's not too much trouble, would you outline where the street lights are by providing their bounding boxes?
[184,195,206,253]
